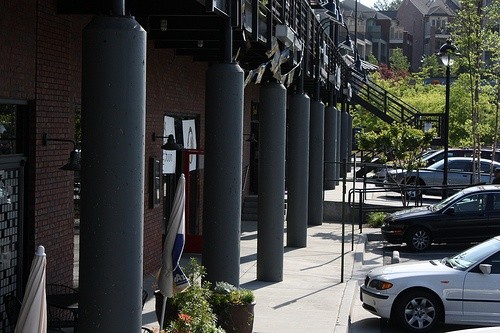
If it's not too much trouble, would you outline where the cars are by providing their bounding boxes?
[359,236,500,333]
[378,147,500,181]
[381,184,500,254]
[384,157,500,199]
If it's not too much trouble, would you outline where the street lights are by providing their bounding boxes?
[435,37,462,200]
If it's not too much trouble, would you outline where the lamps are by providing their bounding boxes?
[42,134,80,172]
[152,133,181,150]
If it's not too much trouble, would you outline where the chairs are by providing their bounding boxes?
[5,284,79,333]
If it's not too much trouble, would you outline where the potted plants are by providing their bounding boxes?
[153,257,256,333]
